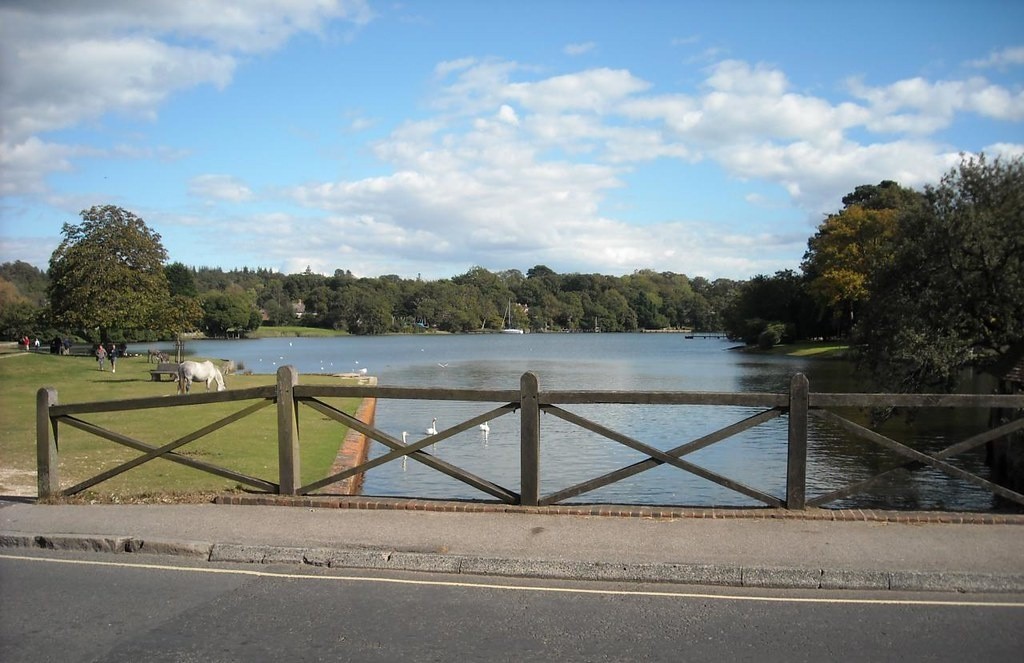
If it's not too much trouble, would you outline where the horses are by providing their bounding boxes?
[175,360,227,395]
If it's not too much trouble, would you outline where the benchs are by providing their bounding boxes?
[149,364,180,382]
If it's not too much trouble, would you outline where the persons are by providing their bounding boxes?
[17,336,30,351]
[33,337,40,353]
[95,344,107,371]
[52,335,69,356]
[108,344,120,374]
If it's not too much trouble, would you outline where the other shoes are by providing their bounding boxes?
[112,369,115,373]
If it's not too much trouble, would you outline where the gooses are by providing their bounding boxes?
[425,417,438,435]
[480,421,489,431]
[389,431,410,451]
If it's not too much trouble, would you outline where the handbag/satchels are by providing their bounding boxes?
[96,356,98,361]
[108,355,112,360]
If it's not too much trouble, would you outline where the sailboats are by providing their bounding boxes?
[499,297,524,335]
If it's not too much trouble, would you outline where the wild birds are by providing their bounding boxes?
[259,340,450,374]
